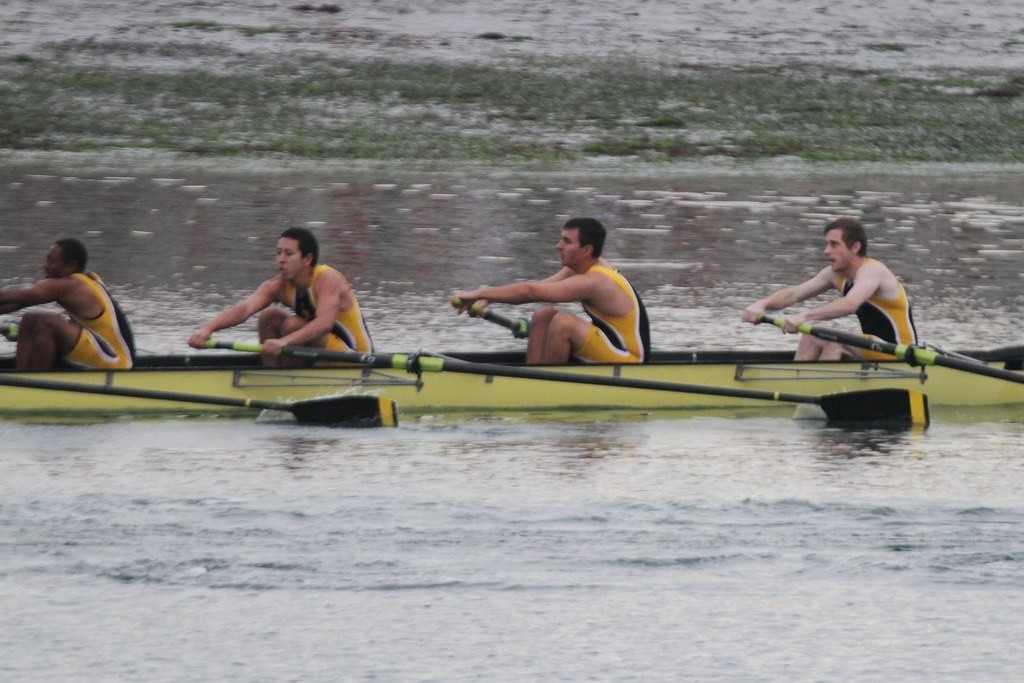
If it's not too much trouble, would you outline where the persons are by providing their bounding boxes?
[450,218,651,365]
[188,227,374,367]
[0,238,136,376]
[745,218,913,362]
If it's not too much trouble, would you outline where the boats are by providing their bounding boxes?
[0,351,1024,412]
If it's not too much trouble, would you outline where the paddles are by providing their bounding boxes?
[199,336,931,429]
[452,297,530,341]
[0,374,399,428]
[756,315,1024,386]
[0,295,169,356]
